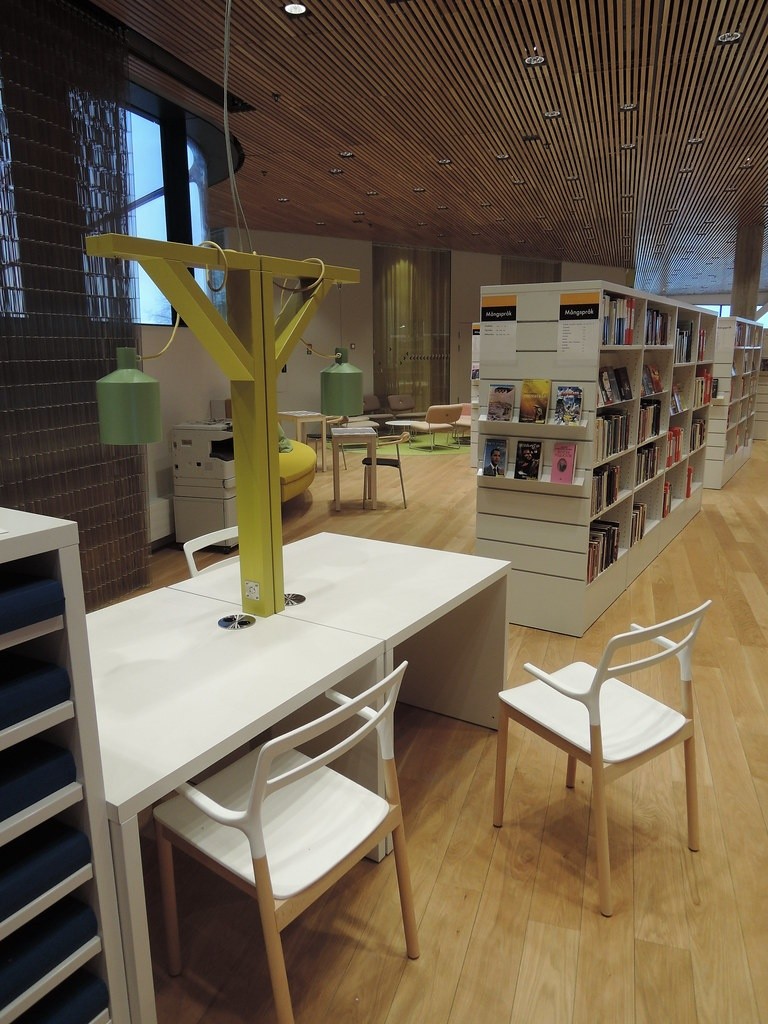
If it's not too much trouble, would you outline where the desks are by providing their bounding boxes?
[83,529,512,1024]
[331,427,377,511]
[385,421,418,435]
[278,411,326,472]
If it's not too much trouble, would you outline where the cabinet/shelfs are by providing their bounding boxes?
[472,280,720,636]
[0,505,133,1024]
[705,315,763,491]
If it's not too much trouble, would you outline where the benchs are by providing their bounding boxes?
[278,440,316,501]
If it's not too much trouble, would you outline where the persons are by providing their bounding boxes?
[517,449,540,475]
[483,447,504,477]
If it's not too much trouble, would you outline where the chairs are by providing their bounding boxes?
[493,604,712,915]
[183,526,242,579]
[447,404,473,442]
[410,404,464,451]
[388,395,428,433]
[154,660,410,1024]
[361,396,396,436]
[361,432,410,509]
[305,416,349,470]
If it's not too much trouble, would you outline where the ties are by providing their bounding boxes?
[493,468,496,476]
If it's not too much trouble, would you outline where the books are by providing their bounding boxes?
[587,295,758,583]
[518,379,552,425]
[483,438,509,477]
[514,441,543,482]
[554,386,583,426]
[486,384,515,422]
[551,443,578,485]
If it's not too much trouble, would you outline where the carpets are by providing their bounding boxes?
[326,430,473,454]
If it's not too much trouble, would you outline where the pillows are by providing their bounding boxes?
[278,424,290,452]
